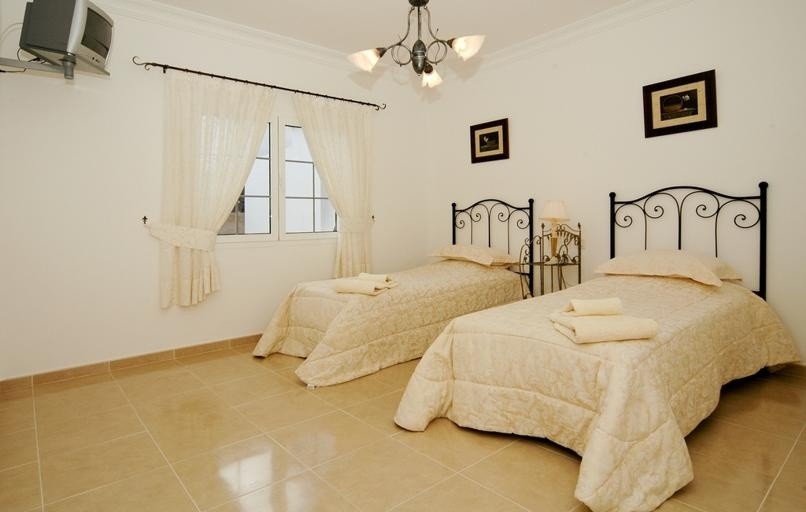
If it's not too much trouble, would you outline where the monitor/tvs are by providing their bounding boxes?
[19,0,114,77]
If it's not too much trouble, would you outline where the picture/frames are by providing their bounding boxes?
[642,69,717,139]
[470,119,509,165]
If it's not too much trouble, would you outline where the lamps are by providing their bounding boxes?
[347,0,487,88]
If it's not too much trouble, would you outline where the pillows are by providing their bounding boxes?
[427,244,518,267]
[592,241,743,288]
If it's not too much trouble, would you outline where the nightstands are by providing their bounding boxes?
[519,219,581,300]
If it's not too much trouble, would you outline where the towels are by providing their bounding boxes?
[548,298,657,345]
[334,272,394,296]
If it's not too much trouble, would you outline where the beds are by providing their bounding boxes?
[253,197,535,386]
[394,182,800,512]
